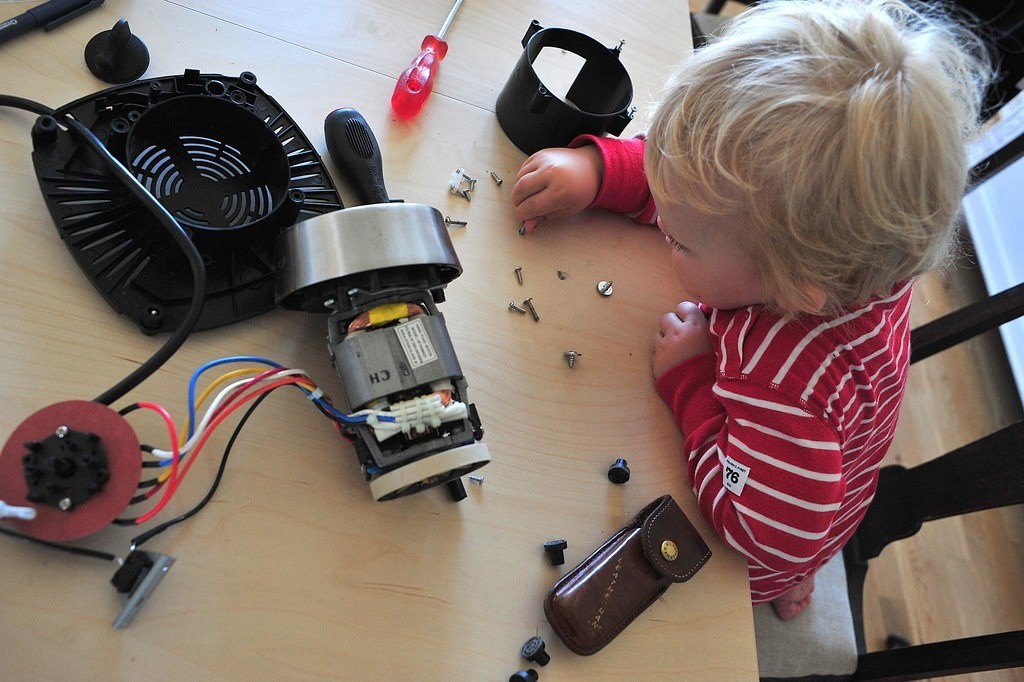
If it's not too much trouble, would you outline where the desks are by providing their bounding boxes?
[1,0,761,682]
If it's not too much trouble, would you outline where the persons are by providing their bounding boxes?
[511,0,999,625]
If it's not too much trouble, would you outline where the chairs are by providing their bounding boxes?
[762,281,1024,682]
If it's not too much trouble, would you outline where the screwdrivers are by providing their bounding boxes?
[387,0,475,125]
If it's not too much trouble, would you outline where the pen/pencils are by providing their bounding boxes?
[0,0,107,49]
